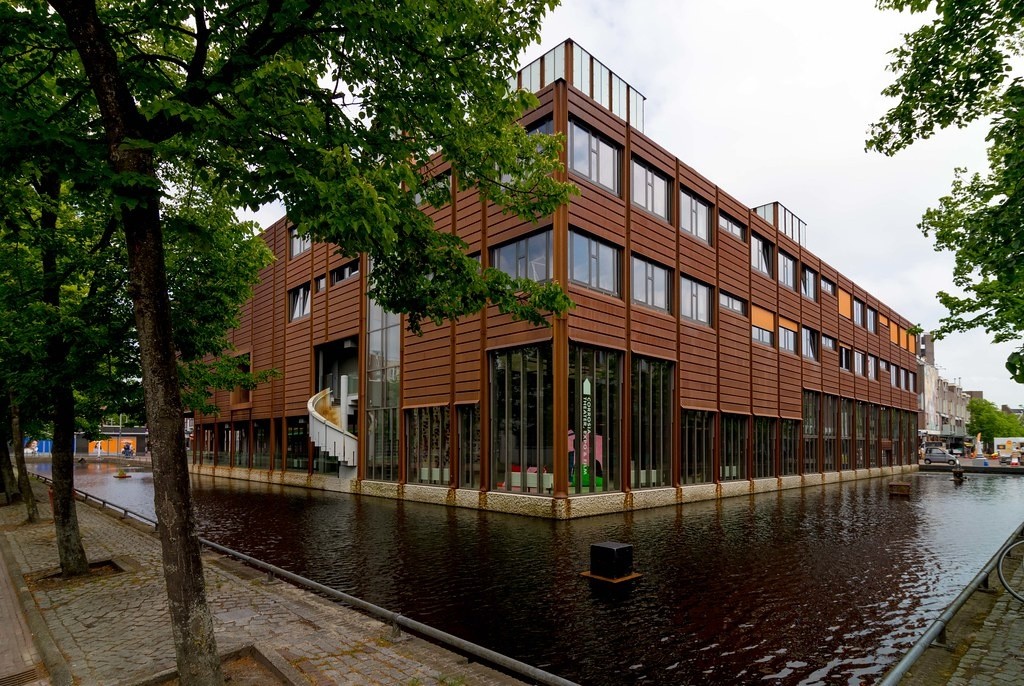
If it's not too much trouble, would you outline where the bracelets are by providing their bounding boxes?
[34,451,38,453]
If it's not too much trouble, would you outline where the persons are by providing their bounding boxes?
[24,438,39,454]
[124,442,134,459]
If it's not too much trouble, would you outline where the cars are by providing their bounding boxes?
[924,447,956,465]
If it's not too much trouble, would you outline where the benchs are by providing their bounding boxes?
[720,466,737,480]
[632,470,657,487]
[421,468,450,485]
[510,472,554,493]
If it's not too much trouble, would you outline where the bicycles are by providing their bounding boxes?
[997,539,1024,603]
